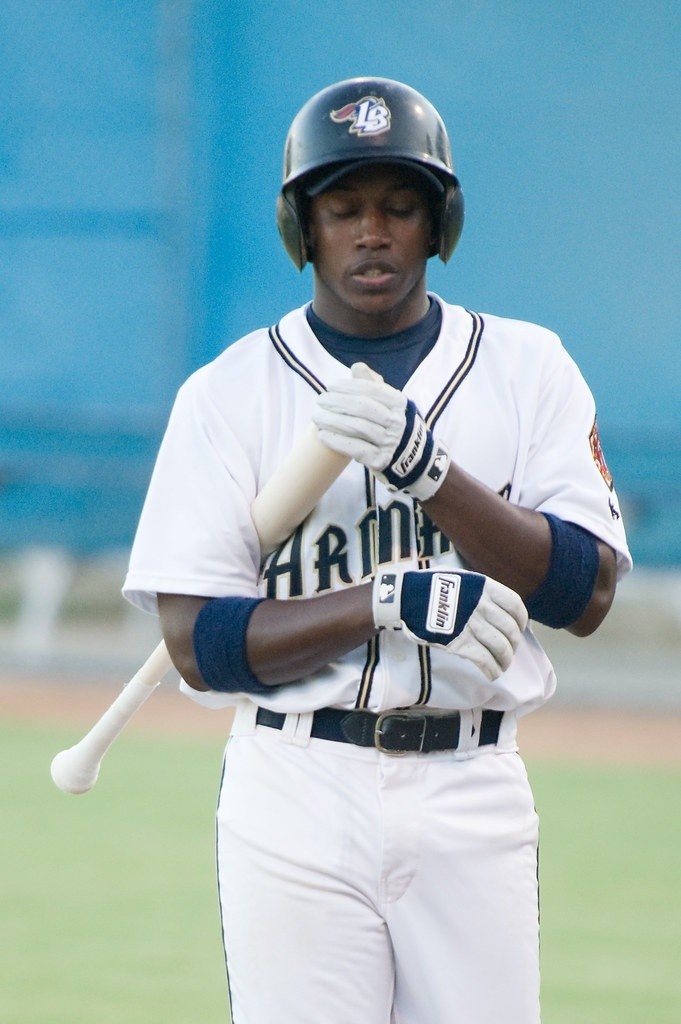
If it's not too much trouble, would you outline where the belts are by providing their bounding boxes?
[256,707,504,757]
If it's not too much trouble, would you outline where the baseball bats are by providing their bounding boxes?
[49,395,363,790]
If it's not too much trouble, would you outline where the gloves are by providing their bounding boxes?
[312,362,451,502]
[372,564,528,682]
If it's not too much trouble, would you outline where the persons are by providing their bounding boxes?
[120,78,632,1024]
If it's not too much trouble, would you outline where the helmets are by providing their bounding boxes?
[276,77,465,273]
